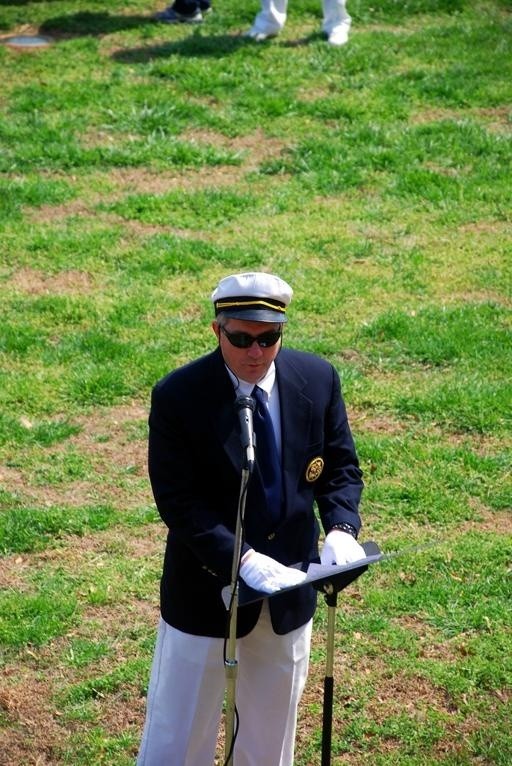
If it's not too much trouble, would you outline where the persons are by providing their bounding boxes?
[137,268,365,766]
[245,0,354,48]
[150,0,216,26]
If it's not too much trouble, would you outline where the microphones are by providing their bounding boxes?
[238,392,259,467]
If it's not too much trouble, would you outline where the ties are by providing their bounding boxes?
[251,385,283,520]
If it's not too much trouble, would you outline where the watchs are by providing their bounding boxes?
[331,522,358,539]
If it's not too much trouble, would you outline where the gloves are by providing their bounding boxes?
[239,548,307,595]
[320,530,366,566]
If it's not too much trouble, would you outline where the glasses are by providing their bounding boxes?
[220,325,282,348]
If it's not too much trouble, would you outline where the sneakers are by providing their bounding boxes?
[246,25,281,42]
[328,23,349,45]
[153,6,204,23]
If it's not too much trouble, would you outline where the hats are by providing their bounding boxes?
[212,272,294,323]
